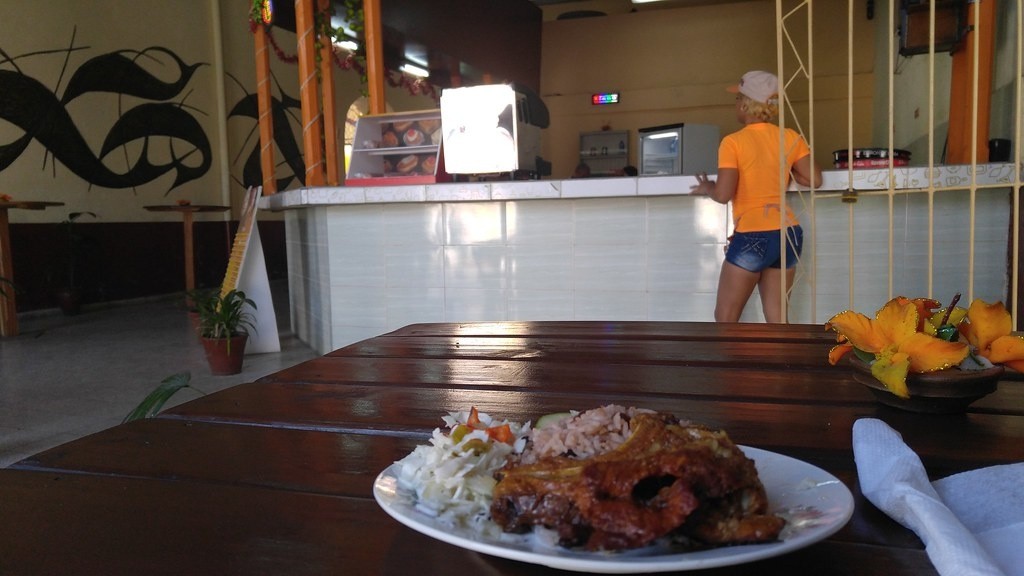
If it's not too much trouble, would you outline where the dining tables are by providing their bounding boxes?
[1,318,1024,576]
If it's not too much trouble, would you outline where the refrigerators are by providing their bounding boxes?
[639,123,722,176]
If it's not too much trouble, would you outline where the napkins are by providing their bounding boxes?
[852,418,1024,576]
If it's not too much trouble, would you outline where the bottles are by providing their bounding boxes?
[620,141,624,152]
[602,144,607,155]
[591,144,596,156]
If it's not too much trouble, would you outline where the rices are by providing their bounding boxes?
[518,404,659,463]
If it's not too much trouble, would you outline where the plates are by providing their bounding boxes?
[373,445,855,573]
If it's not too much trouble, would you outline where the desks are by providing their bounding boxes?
[0,200,65,340]
[142,202,232,312]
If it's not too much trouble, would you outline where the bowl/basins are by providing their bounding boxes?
[848,352,1006,411]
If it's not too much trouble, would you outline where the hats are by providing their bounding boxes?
[726,71,778,104]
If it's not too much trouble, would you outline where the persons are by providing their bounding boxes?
[569,155,590,178]
[623,166,638,177]
[689,71,822,323]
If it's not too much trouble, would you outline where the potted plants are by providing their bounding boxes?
[181,282,259,376]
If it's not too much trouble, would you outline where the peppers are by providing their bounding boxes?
[450,406,516,454]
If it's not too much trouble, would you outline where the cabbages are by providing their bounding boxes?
[393,411,532,536]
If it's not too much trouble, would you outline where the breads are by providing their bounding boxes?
[380,119,440,176]
